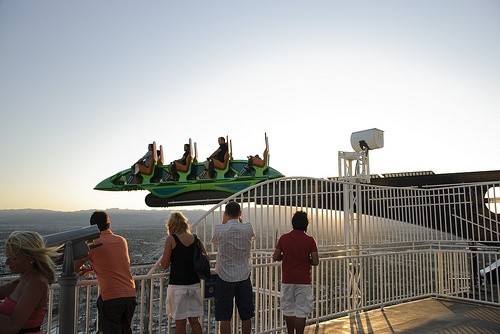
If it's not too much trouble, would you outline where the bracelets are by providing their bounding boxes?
[88,265,92,270]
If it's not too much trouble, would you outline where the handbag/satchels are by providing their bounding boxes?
[193,234,211,280]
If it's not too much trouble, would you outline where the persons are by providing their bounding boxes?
[207,137,228,178]
[167,143,190,181]
[157,150,160,160]
[210,201,256,334]
[161,213,205,334]
[73,210,136,334]
[133,143,153,183]
[273,210,320,334]
[0,231,65,334]
